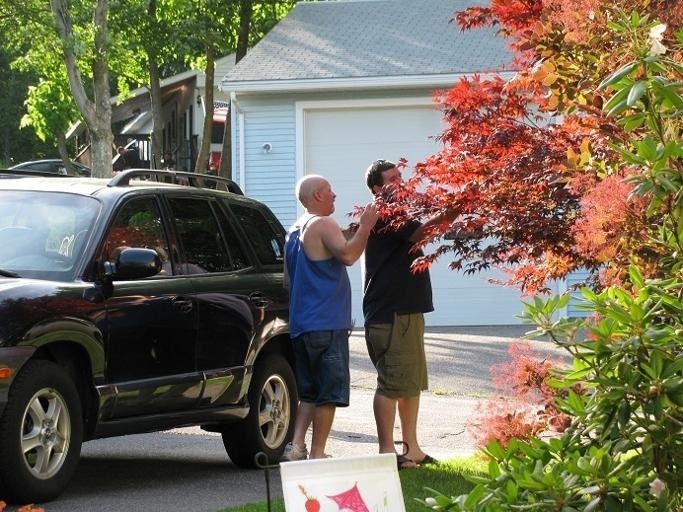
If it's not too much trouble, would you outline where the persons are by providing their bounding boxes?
[283,176,381,460]
[118,147,139,168]
[364,161,438,468]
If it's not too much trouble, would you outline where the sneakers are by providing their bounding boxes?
[283,443,308,462]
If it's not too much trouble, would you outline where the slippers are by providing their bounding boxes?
[397,455,443,468]
